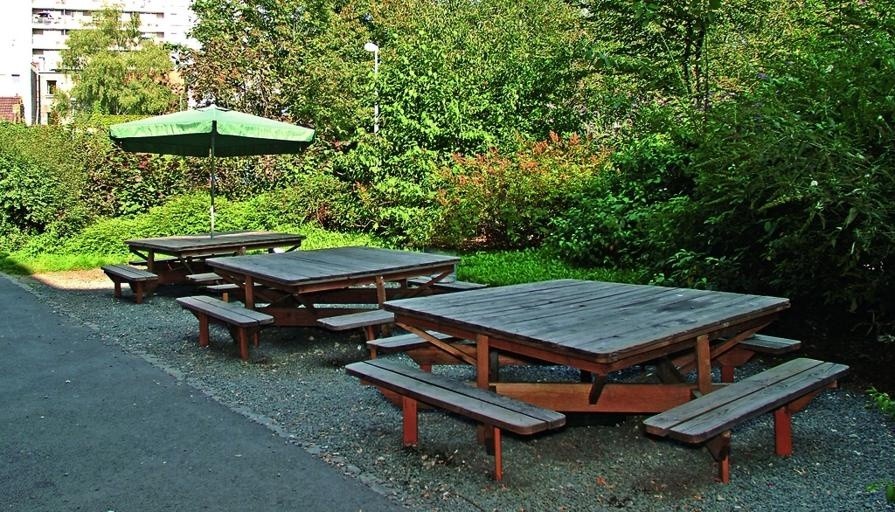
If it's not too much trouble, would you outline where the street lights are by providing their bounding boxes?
[363,43,380,132]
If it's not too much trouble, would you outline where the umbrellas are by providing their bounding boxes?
[107,100,317,241]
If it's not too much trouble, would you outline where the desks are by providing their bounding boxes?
[208,249,461,299]
[384,280,792,444]
[123,230,306,303]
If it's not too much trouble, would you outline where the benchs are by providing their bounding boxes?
[643,356,852,483]
[344,358,568,481]
[103,262,160,304]
[210,281,261,302]
[176,293,274,358]
[187,273,221,284]
[718,332,802,383]
[367,328,464,372]
[315,308,395,345]
